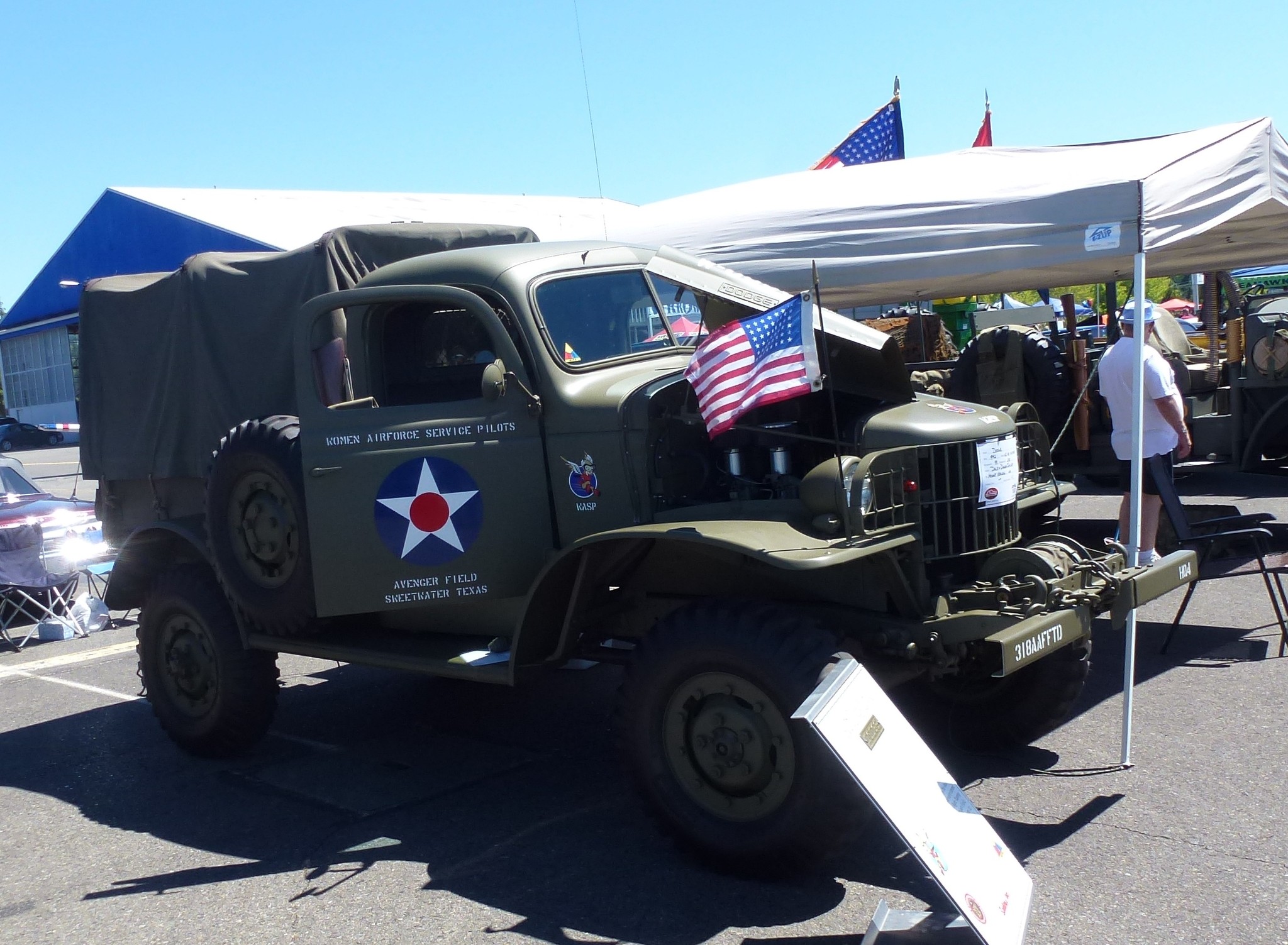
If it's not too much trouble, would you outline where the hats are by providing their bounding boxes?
[1119,300,1162,324]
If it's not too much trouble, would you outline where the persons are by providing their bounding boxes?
[1180,307,1195,319]
[1098,298,1191,567]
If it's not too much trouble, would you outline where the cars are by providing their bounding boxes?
[1041,317,1204,338]
[0,454,118,609]
[0,417,65,452]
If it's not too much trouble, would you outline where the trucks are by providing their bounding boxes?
[71,226,1201,881]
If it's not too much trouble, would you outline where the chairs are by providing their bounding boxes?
[1146,452,1288,658]
[0,524,141,652]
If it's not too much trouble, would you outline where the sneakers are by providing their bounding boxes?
[1139,548,1160,565]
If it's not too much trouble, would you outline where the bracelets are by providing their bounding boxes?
[1178,427,1189,437]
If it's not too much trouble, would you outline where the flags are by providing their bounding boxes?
[971,112,992,149]
[684,289,825,433]
[811,99,906,171]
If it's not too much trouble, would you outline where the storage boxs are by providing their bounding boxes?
[933,302,978,351]
[38,620,75,640]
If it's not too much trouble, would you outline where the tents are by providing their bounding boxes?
[1157,299,1203,316]
[990,294,1031,310]
[557,111,1288,770]
[1033,296,1094,318]
[642,314,708,343]
[1190,263,1288,315]
